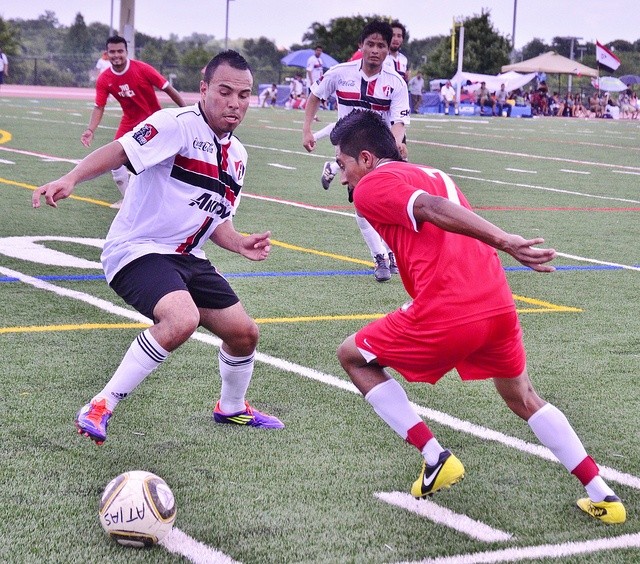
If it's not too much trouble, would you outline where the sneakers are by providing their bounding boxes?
[373,246,391,281]
[576,495,626,523]
[388,252,399,274]
[75,397,113,445]
[214,400,285,429]
[321,161,336,190]
[410,449,465,501]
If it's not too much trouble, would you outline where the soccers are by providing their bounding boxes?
[99,469,178,548]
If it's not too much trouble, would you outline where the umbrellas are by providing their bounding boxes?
[590,75,627,94]
[281,47,338,71]
[618,74,640,85]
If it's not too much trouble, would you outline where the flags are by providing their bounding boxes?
[595,40,622,72]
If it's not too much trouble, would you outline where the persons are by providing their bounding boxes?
[289,72,304,103]
[477,82,495,116]
[294,94,302,108]
[0,50,9,77]
[95,51,111,74]
[329,106,626,523]
[259,80,279,108]
[79,37,185,211]
[590,93,604,117]
[496,83,512,117]
[537,80,548,115]
[405,69,426,113]
[629,90,640,120]
[303,22,409,280]
[307,45,325,124]
[523,92,529,103]
[550,91,565,117]
[31,49,284,445]
[441,78,460,117]
[575,93,585,112]
[351,19,408,76]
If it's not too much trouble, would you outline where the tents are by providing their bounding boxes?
[502,51,600,100]
[449,70,534,96]
